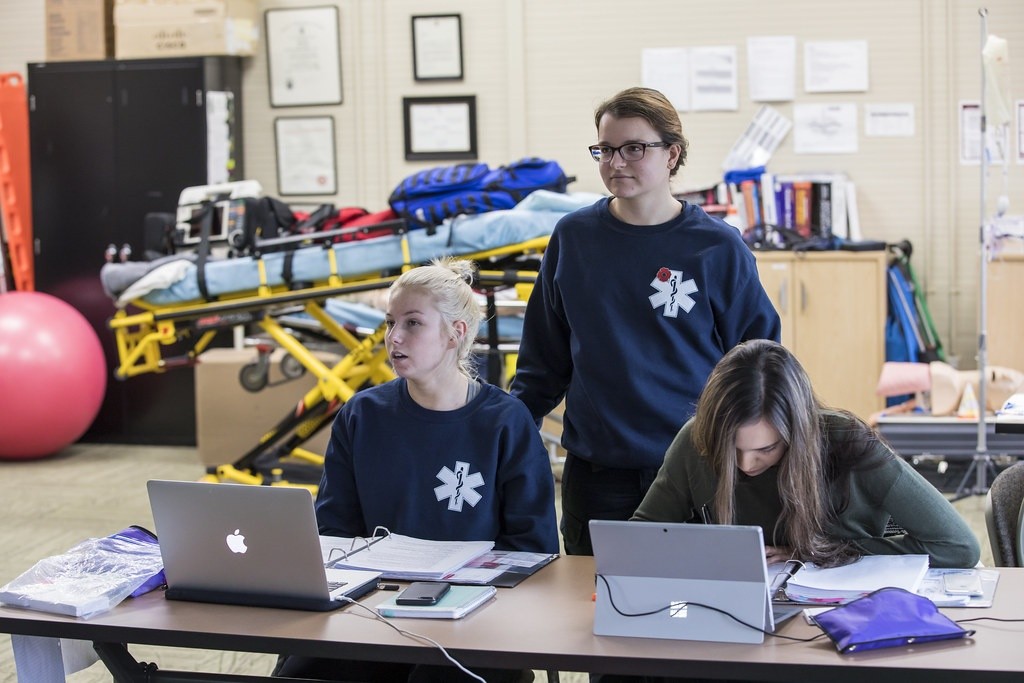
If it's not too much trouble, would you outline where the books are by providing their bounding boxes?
[702,171,857,241]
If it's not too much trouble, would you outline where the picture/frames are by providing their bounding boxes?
[403,96,479,161]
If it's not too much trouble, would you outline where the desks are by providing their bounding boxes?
[0,535,1024,683]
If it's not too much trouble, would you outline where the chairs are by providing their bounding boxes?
[986,461,1024,567]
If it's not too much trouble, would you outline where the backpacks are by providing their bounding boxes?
[294,204,400,241]
[247,196,300,251]
[389,159,577,235]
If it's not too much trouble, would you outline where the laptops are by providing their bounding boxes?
[145,479,383,611]
[589,519,802,634]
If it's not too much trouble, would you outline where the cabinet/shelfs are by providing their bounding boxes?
[747,250,889,430]
[26,54,244,445]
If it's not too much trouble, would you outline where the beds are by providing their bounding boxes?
[100,185,605,378]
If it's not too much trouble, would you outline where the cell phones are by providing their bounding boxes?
[395,582,450,606]
[943,570,984,596]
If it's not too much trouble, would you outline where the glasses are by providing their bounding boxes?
[588,140,671,164]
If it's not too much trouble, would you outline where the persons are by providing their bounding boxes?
[271,254,559,683]
[627,338,981,683]
[510,87,780,683]
